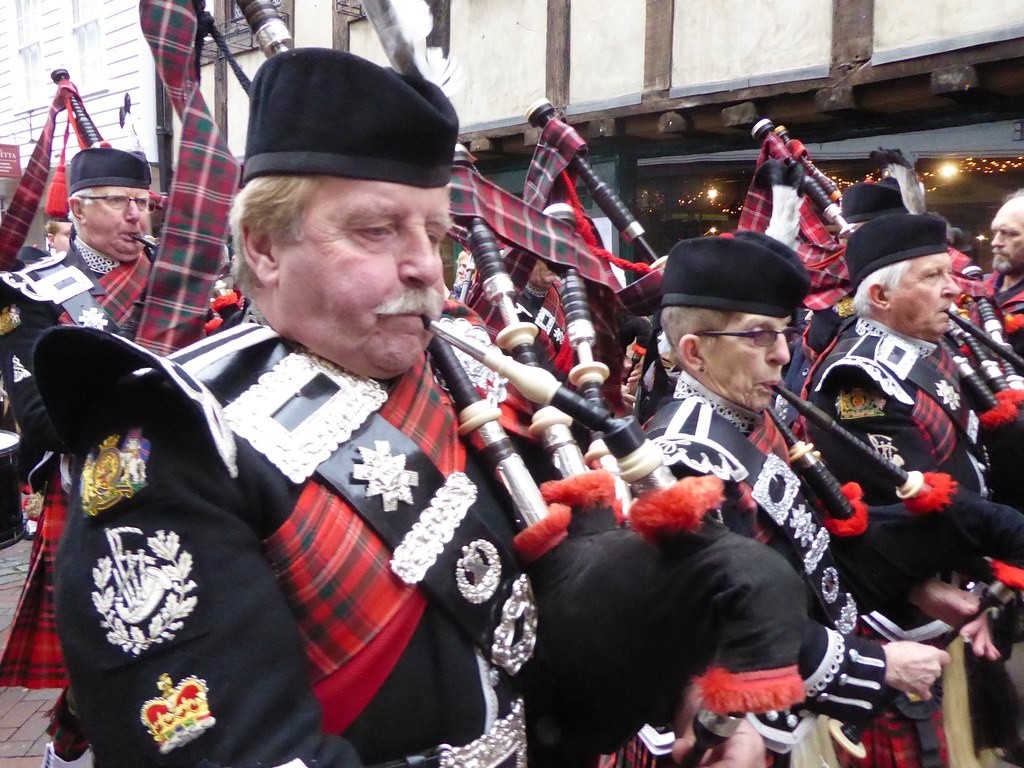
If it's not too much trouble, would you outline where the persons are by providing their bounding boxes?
[44,218,73,254]
[512,254,621,414]
[799,212,1024,768]
[982,190,1024,356]
[842,177,1007,389]
[30,48,589,768]
[639,232,948,768]
[449,248,477,300]
[0,146,244,768]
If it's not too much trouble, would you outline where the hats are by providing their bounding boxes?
[70,148,151,195]
[846,213,948,288]
[661,231,811,317]
[839,178,910,223]
[242,48,462,186]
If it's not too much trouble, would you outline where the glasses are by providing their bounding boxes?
[77,194,156,214]
[693,326,803,348]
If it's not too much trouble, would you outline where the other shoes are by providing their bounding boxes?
[40,741,95,768]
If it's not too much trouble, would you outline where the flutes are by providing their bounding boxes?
[521,96,1024,758]
[752,112,1024,439]
[46,64,241,339]
[236,0,811,758]
